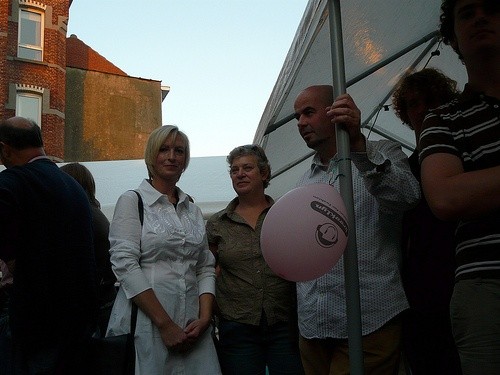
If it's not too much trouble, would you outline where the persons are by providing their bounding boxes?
[205,145,305,375]
[106,125,223,375]
[394,0,500,375]
[0,117,110,375]
[294,85,422,375]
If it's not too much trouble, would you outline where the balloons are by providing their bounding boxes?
[260,183,349,283]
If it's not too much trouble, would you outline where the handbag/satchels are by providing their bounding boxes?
[73,332,137,375]
